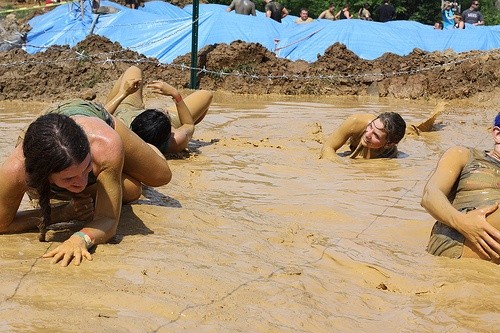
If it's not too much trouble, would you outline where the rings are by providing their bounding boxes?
[83,204,88,209]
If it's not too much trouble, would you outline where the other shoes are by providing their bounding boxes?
[92,0,100,13]
[98,6,119,13]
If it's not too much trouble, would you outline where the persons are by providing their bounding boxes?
[226,0,256,16]
[103,66,213,156]
[431,0,485,30]
[0,100,172,267]
[295,6,314,23]
[335,4,353,20]
[320,112,406,164]
[371,0,397,23]
[318,2,336,21]
[262,0,289,23]
[358,2,373,21]
[420,111,500,264]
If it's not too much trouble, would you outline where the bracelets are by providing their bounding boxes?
[171,93,182,103]
[72,229,95,249]
